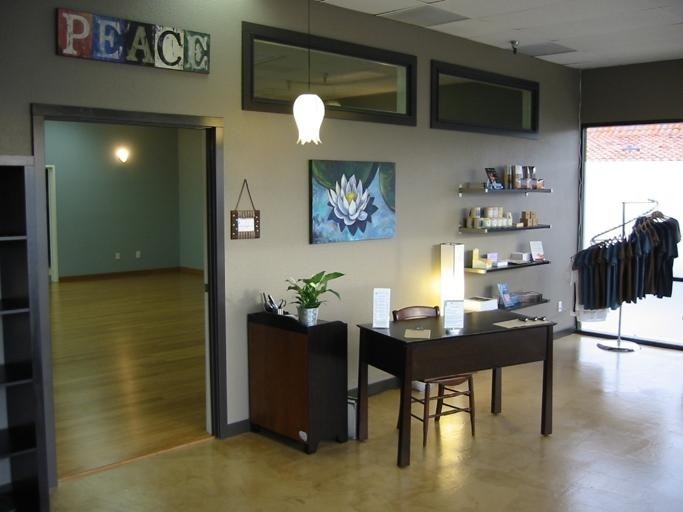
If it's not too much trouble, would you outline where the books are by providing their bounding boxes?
[504,164,523,189]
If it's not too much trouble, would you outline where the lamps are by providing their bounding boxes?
[114,147,130,163]
[440,242,464,317]
[291,0,325,146]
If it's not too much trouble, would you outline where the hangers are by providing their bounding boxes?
[589,196,658,353]
[567,211,671,266]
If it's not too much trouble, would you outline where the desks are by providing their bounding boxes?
[355,309,557,470]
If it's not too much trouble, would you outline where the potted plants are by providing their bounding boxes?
[285,269,344,326]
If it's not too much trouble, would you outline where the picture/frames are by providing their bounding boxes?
[229,177,261,238]
[307,159,395,244]
[54,6,211,75]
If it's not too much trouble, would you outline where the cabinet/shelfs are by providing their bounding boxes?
[45,163,59,283]
[457,187,550,309]
[246,310,348,455]
[0,154,52,512]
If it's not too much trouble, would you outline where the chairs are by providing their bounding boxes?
[391,305,475,448]
